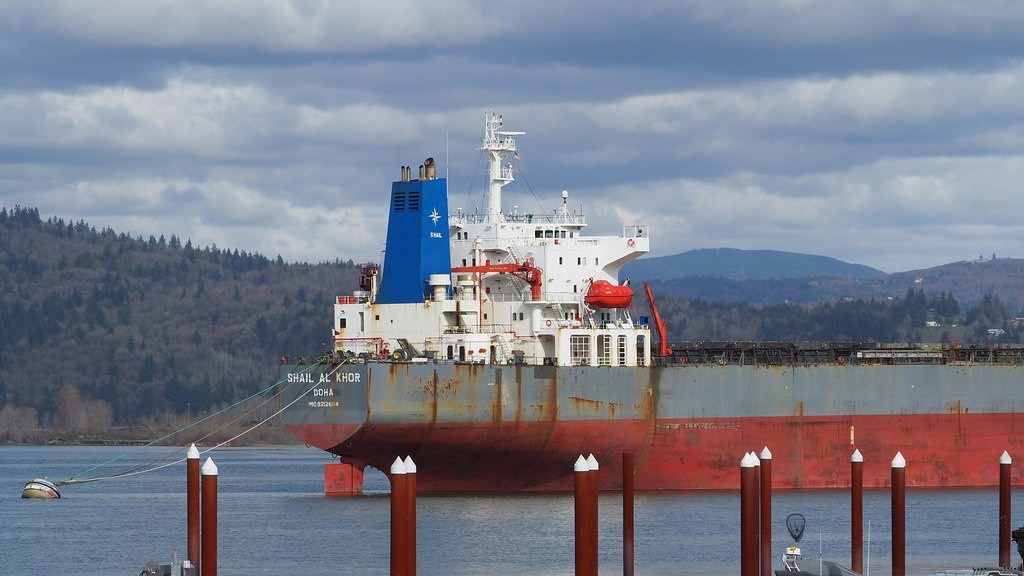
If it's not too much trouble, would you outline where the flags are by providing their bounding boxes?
[514,151,520,160]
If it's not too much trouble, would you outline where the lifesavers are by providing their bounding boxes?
[628,238,634,246]
[837,356,845,366]
[546,319,552,326]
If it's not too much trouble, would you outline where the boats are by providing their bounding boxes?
[774,514,1024,576]
[278,108,1024,493]
